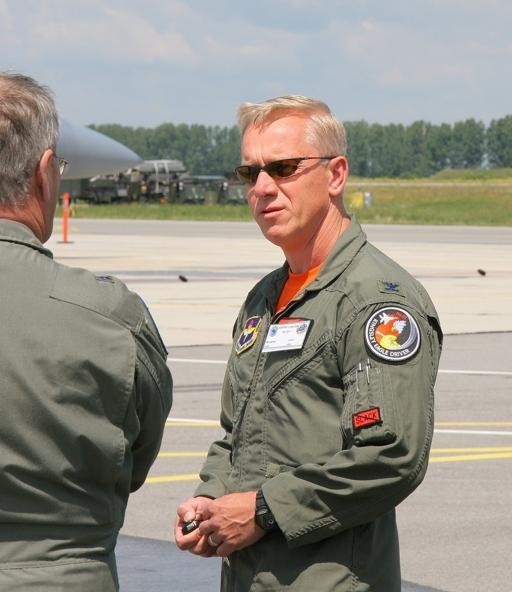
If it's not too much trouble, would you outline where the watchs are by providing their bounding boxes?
[254,488,278,532]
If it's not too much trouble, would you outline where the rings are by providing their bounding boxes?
[208,534,221,547]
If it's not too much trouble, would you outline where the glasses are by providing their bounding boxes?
[234,156,334,181]
[53,155,68,176]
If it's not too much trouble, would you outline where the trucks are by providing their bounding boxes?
[80,160,250,205]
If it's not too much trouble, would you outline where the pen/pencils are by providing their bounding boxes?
[355,358,371,393]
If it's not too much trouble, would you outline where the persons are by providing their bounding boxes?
[172,91,444,591]
[1,72,173,590]
